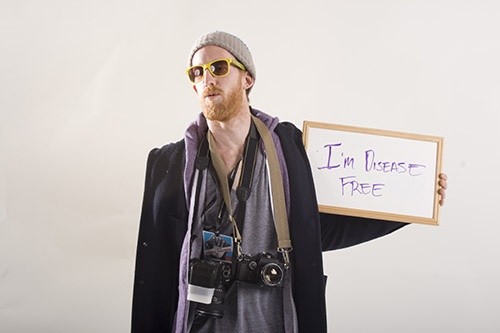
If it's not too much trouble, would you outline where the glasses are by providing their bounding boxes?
[187,58,245,84]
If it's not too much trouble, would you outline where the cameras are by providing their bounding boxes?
[233,252,290,288]
[190,258,232,320]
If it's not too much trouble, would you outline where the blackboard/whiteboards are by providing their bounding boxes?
[302,121,445,225]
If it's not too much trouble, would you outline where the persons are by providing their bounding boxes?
[131,30,450,333]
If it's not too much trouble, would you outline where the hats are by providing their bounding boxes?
[188,30,255,94]
